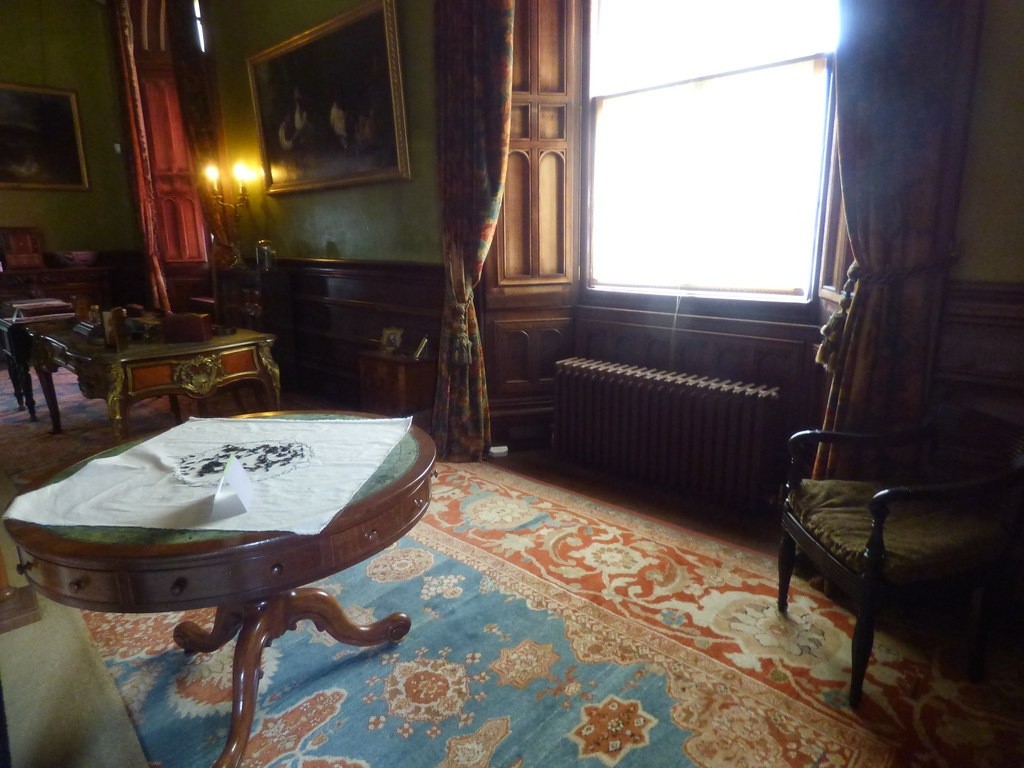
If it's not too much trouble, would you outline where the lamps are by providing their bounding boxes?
[201,160,258,270]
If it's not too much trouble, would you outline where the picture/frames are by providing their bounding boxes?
[244,0,414,198]
[0,80,92,193]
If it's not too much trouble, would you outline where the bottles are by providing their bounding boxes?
[88,305,99,320]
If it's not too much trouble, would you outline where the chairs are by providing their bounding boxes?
[774,400,1024,711]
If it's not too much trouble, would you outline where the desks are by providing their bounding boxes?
[27,311,283,444]
[6,406,437,768]
[0,306,78,422]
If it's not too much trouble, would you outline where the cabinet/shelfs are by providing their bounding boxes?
[0,264,117,321]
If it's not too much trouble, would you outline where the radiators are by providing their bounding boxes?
[550,355,780,528]
